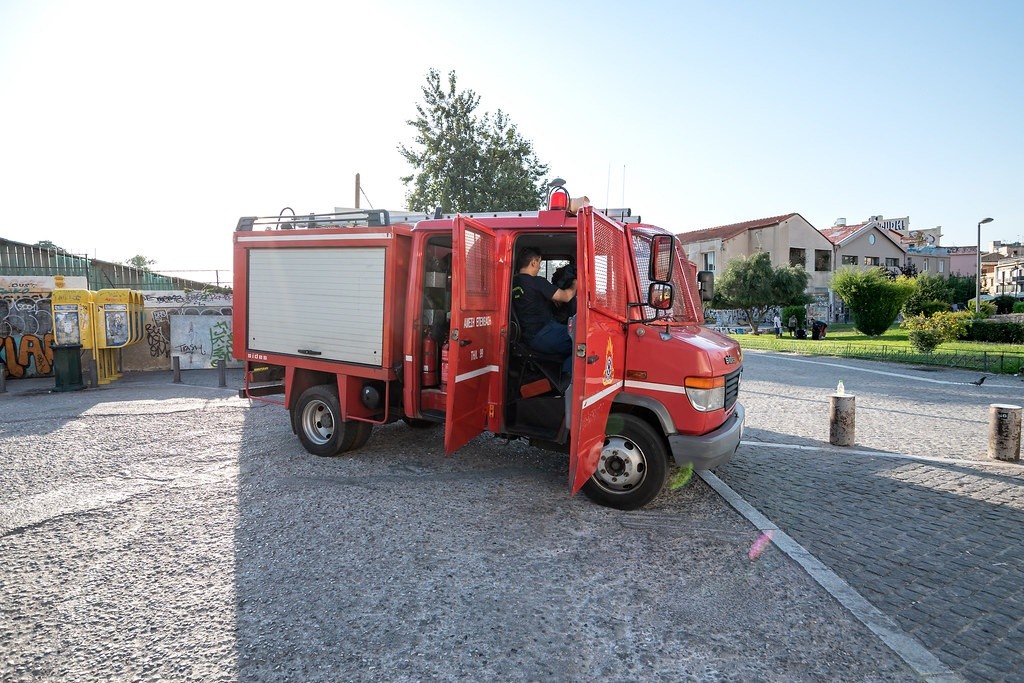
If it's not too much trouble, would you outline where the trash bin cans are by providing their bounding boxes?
[49,343,84,393]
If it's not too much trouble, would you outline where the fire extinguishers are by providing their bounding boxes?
[422,328,439,387]
[440,332,449,393]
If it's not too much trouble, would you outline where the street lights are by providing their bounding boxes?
[976,218,994,315]
[547,180,566,210]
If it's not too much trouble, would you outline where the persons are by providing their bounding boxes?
[774,313,783,339]
[513,246,578,376]
[811,318,827,340]
[788,315,807,339]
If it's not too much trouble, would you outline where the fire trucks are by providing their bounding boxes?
[229,186,746,512]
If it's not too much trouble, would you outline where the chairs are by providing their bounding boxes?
[510,309,557,362]
